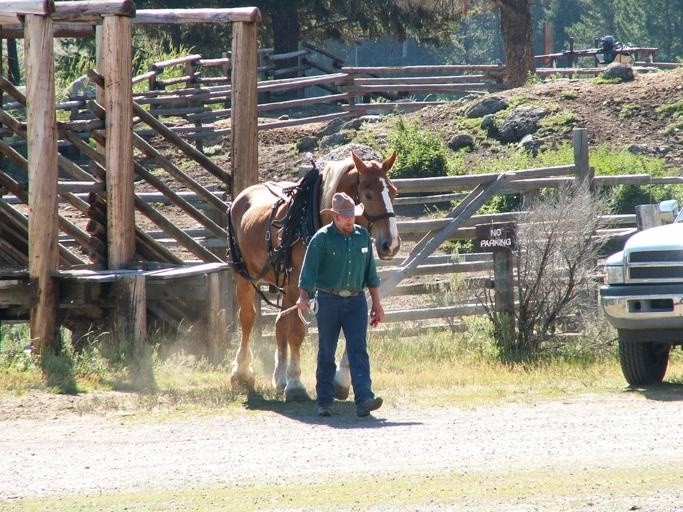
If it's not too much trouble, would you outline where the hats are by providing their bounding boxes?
[320,192,364,217]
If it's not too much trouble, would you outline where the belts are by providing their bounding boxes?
[317,288,360,297]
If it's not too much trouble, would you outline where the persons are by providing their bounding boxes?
[293,192,384,420]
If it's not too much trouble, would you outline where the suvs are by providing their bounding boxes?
[598,199,682,386]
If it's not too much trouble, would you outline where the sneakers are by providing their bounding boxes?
[319,408,331,414]
[358,398,384,417]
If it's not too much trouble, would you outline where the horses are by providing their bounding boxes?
[225,149,402,403]
